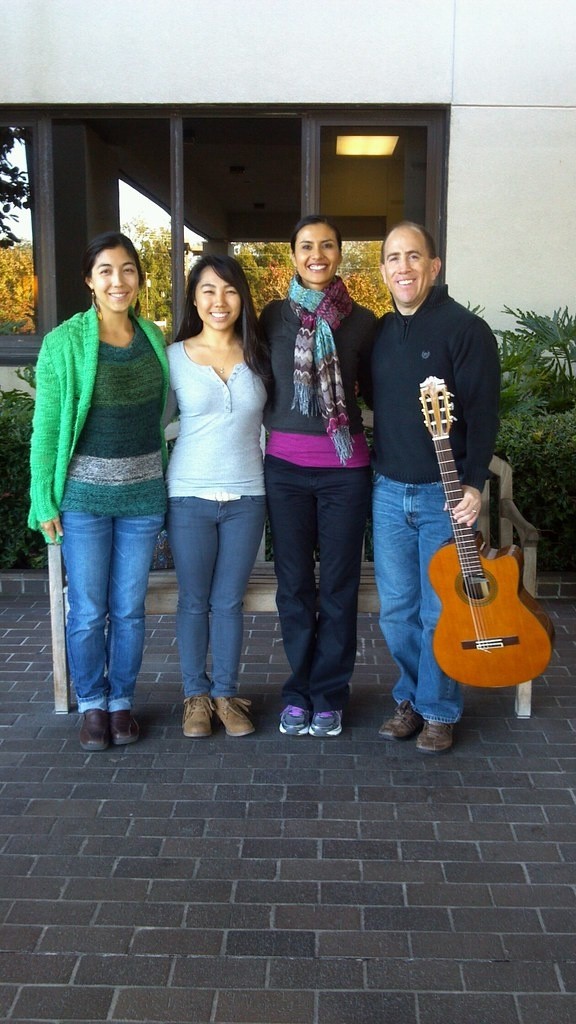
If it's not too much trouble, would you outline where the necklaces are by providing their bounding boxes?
[198,330,238,376]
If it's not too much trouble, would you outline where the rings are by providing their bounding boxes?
[471,510,477,514]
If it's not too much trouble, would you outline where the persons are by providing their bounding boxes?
[27,212,502,754]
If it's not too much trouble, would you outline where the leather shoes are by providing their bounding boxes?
[79,709,111,750]
[110,710,139,745]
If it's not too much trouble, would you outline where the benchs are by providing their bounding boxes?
[44,451,540,719]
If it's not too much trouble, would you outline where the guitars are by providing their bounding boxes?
[419,374,555,687]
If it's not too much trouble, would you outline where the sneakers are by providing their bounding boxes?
[377,700,424,741]
[309,709,344,738]
[182,693,216,738]
[416,720,455,754]
[278,704,312,736]
[212,696,255,736]
